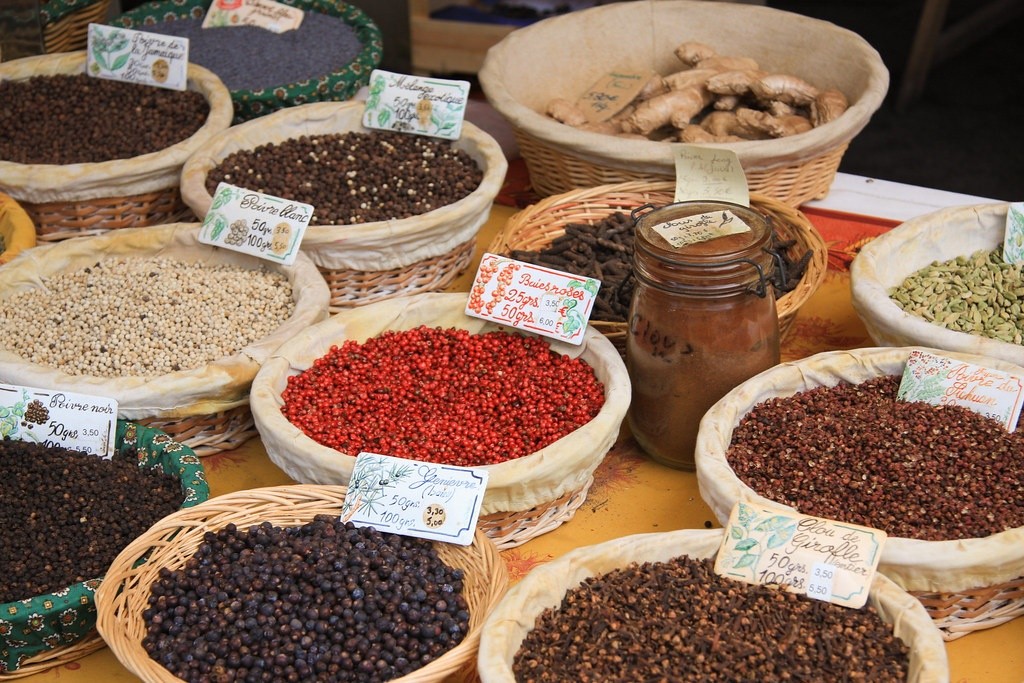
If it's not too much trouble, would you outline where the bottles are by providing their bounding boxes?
[624,200,787,469]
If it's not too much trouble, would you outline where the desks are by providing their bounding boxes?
[0,198,1024,683]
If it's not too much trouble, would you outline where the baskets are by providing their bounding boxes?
[472,528,948,683]
[478,0,890,210]
[486,180,828,371]
[104,0,382,130]
[0,0,105,62]
[180,101,509,311]
[851,202,1024,367]
[0,51,235,235]
[0,422,211,680]
[0,224,332,457]
[696,346,1024,641]
[252,292,631,550]
[94,485,507,683]
